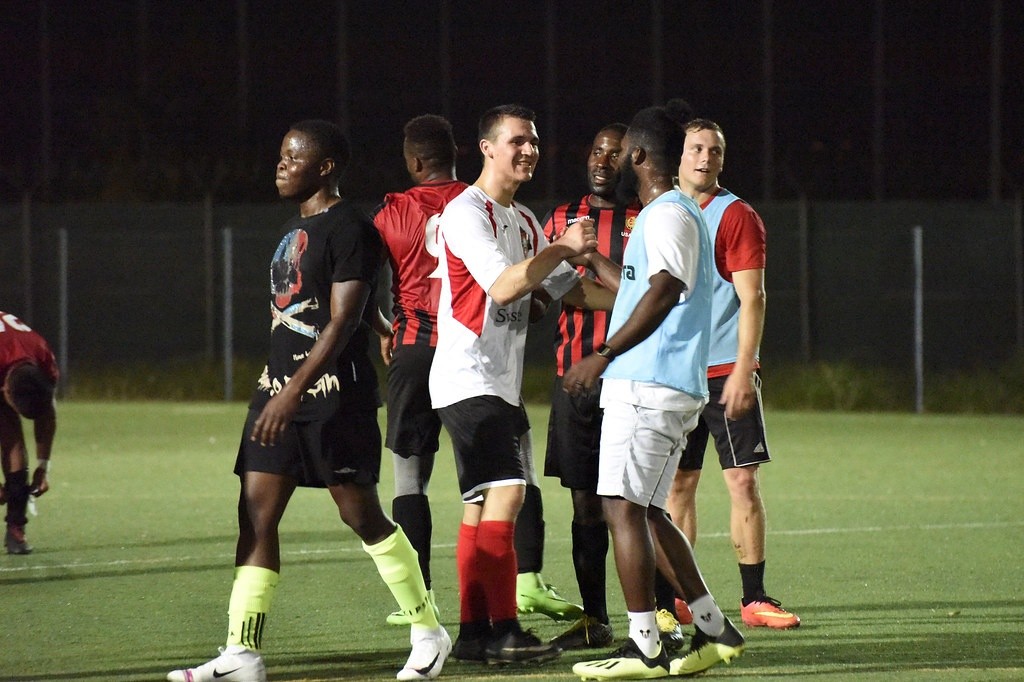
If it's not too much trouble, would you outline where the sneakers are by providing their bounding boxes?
[386,606,439,625]
[551,614,614,650]
[572,637,670,682]
[167,646,267,682]
[655,609,685,650]
[397,624,452,681]
[453,631,562,668]
[669,615,745,676]
[674,598,693,625]
[516,585,584,619]
[741,598,800,631]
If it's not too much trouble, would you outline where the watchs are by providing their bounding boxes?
[594,342,615,363]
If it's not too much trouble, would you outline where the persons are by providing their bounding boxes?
[528,124,684,652]
[552,108,746,681]
[428,104,616,667]
[0,310,60,556]
[166,122,453,682]
[666,117,801,629]
[371,114,584,626]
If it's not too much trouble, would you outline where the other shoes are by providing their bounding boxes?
[4,523,34,554]
